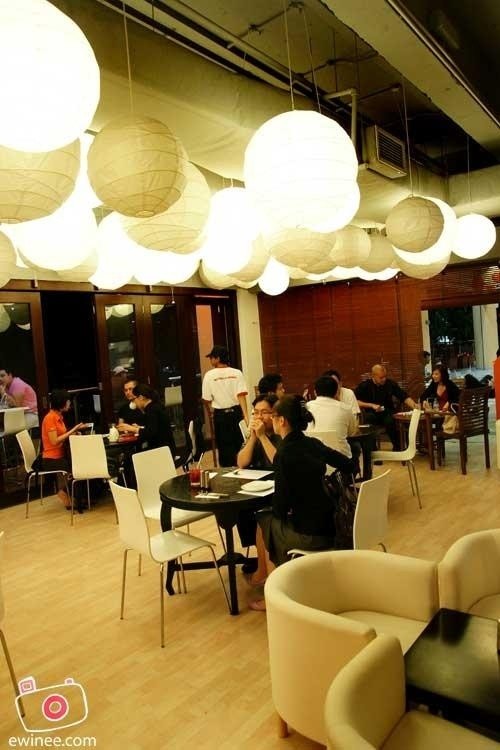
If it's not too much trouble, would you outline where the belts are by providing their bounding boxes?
[214,406,240,413]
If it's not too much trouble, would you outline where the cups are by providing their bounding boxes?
[188,464,202,487]
[129,402,137,411]
[109,428,120,443]
[426,403,432,412]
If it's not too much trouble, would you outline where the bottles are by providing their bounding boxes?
[2,392,8,409]
[433,398,440,414]
[199,469,210,490]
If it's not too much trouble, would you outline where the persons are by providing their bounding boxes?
[0,368,38,471]
[40,387,82,510]
[115,347,494,613]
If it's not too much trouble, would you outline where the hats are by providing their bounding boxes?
[206,345,229,357]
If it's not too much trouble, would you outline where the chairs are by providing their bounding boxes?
[0,384,500,750]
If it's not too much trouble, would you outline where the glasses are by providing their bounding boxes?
[252,411,270,416]
[270,414,281,420]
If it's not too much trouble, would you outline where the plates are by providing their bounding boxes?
[240,480,273,492]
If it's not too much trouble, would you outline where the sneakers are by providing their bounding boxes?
[247,572,268,587]
[248,597,266,610]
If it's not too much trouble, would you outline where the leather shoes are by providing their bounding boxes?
[242,558,258,574]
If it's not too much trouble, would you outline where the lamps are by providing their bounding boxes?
[0,0,498,297]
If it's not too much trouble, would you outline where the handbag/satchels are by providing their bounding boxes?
[442,403,474,434]
[31,457,67,471]
[325,475,358,543]
